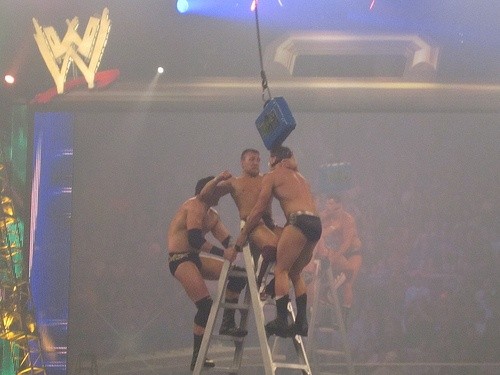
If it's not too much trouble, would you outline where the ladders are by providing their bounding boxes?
[270,253,358,374]
[190,234,314,375]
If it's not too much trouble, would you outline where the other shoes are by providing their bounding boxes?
[287,321,309,337]
[264,319,289,338]
[264,282,275,298]
[191,357,216,370]
[218,323,248,337]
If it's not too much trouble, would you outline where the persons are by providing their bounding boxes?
[224,147,321,337]
[200,149,311,297]
[302,195,362,309]
[168,175,249,371]
